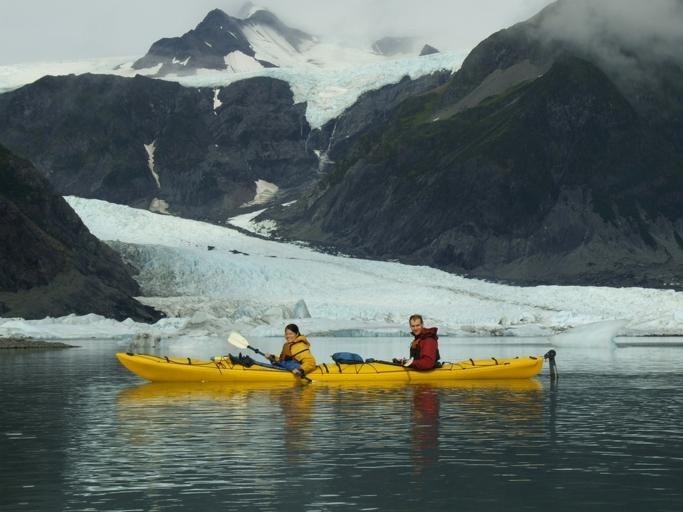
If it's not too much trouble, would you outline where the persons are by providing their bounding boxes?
[271,385,316,467]
[409,387,441,472]
[400,314,440,370]
[265,324,316,377]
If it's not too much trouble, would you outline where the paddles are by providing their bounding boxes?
[393,357,413,367]
[227,332,312,382]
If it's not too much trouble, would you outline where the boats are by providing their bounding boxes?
[117,381,545,406]
[115,350,542,380]
